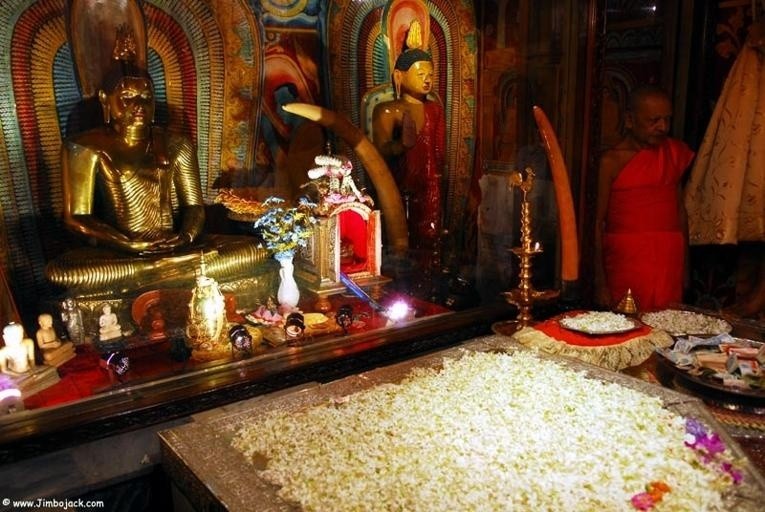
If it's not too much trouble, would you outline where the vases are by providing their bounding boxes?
[275,251,301,308]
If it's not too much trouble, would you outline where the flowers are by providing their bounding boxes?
[254,196,318,254]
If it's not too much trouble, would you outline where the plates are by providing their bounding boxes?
[639,308,735,336]
[558,311,643,338]
[650,333,764,415]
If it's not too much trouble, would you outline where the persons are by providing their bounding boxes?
[596,88,695,312]
[373,19,446,278]
[38,25,279,346]
[1,298,120,406]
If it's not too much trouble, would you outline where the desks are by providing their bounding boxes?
[0,283,488,470]
[158,300,765,512]
